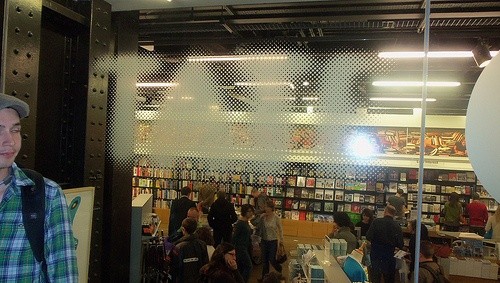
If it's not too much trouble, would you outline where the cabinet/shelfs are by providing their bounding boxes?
[131,154,494,224]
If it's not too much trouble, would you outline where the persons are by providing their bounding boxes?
[465,192,488,237]
[166,184,287,282]
[330,190,446,283]
[441,191,462,232]
[0,94,80,283]
[485,206,500,240]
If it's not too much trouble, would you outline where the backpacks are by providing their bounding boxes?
[418,263,450,283]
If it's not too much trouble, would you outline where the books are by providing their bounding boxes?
[133,161,498,222]
[396,230,500,280]
[142,214,163,241]
[290,239,372,283]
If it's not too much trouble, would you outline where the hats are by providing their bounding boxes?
[0,92,30,120]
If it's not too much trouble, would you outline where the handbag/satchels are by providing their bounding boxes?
[278,245,287,264]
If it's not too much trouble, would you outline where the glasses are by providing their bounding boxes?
[228,253,237,257]
